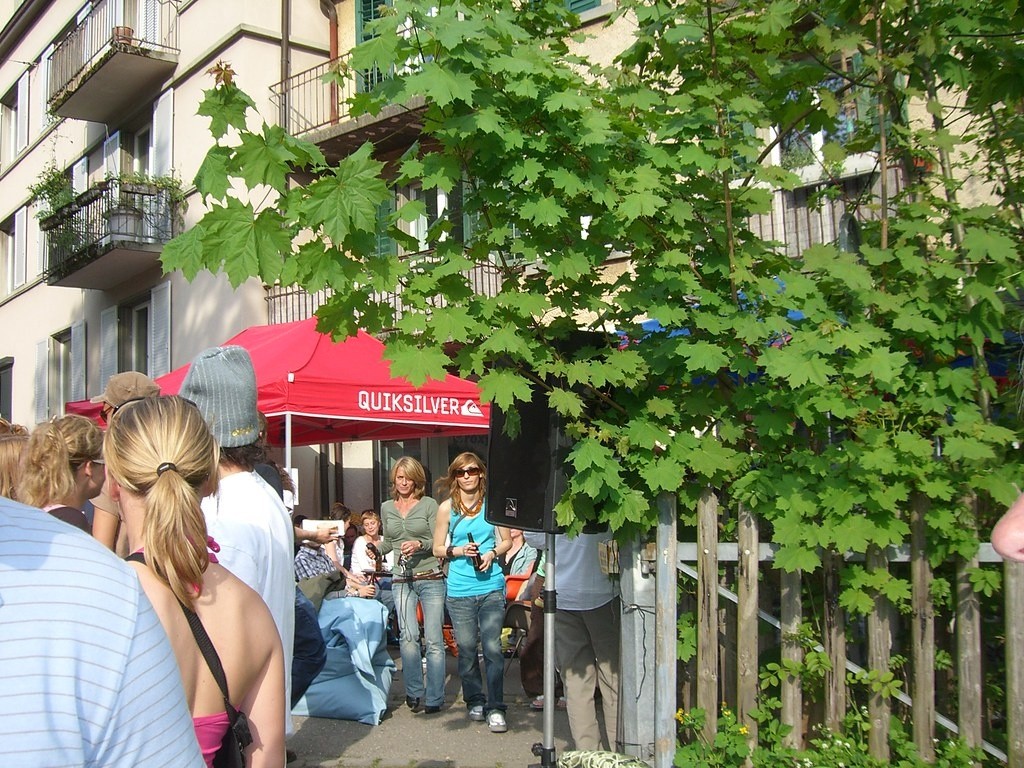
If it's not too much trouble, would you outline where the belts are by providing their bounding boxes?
[392,570,445,583]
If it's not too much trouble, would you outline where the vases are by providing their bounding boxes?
[111,26,134,46]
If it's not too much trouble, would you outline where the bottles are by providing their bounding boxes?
[465,532,484,571]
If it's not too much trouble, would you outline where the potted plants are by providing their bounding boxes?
[102,197,146,242]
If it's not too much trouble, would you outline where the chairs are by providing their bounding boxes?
[501,558,535,600]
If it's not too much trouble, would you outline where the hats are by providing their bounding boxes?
[179,344,261,447]
[89,370,162,409]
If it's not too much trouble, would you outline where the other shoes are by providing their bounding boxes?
[425,705,441,713]
[529,695,566,710]
[469,706,508,733]
[405,694,420,712]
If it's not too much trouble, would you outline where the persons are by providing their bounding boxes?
[0,346,623,768]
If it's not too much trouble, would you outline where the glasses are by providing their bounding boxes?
[99,405,112,423]
[453,467,481,478]
